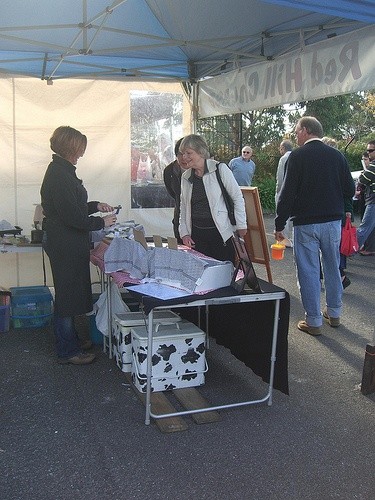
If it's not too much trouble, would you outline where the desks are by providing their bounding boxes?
[91,239,286,427]
[0,239,42,253]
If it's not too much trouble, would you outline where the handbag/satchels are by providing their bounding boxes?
[340,216,359,256]
[95,281,130,337]
[215,162,236,226]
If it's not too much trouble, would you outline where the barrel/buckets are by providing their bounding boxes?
[271,239,285,260]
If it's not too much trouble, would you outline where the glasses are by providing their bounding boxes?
[367,149,375,153]
[242,151,251,154]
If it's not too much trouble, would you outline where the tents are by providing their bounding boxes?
[1,1,375,290]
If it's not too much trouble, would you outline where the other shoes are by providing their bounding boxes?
[298,320,323,335]
[360,250,375,255]
[323,311,340,327]
[342,276,351,289]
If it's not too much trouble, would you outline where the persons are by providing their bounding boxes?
[274,115,355,333]
[229,146,255,186]
[321,137,351,289]
[354,141,375,255]
[41,125,117,366]
[275,140,292,246]
[164,137,191,243]
[179,135,248,258]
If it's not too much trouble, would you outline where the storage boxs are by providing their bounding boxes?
[113,310,209,394]
[9,285,53,329]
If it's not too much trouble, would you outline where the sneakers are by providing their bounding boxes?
[59,352,96,364]
[78,339,93,349]
